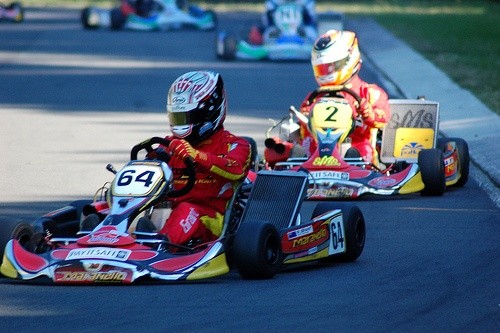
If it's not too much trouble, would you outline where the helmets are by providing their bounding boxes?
[311,30,362,86]
[167,70,228,145]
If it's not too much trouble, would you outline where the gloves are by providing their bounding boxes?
[165,139,199,161]
[354,98,375,123]
[300,101,313,117]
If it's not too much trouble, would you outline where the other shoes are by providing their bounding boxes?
[135,217,157,247]
[81,214,99,236]
[345,147,364,166]
[290,144,308,162]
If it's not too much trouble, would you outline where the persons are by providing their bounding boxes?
[300,29,391,172]
[78,70,253,253]
